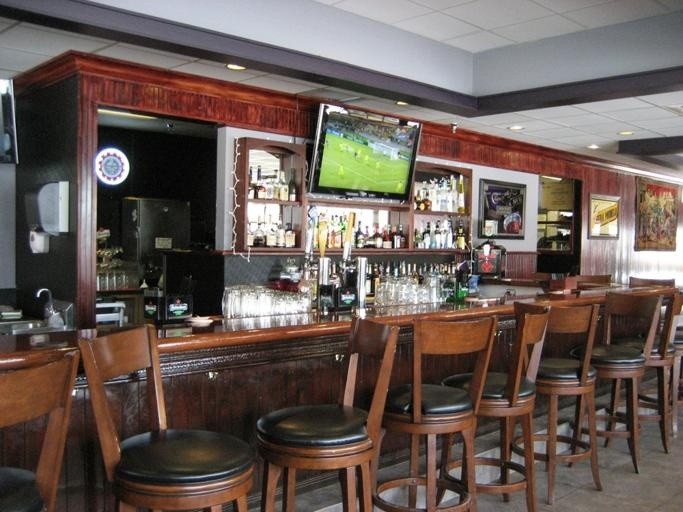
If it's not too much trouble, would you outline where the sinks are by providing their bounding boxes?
[0,319,66,335]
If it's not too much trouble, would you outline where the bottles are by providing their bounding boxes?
[247,165,298,201]
[280,256,359,309]
[365,259,469,307]
[355,220,407,249]
[414,171,465,214]
[237,212,305,253]
[411,215,467,249]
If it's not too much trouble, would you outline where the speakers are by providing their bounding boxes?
[122,196,193,261]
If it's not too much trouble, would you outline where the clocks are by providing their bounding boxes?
[92,147,130,186]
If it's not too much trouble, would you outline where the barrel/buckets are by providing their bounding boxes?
[36,287,57,320]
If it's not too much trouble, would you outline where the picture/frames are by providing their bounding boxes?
[588,193,621,239]
[474,249,501,276]
[478,179,526,240]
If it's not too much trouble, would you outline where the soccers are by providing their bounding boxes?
[382,153,386,157]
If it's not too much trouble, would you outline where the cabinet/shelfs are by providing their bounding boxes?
[537,220,575,255]
[233,136,472,256]
[143,249,198,337]
[95,289,144,335]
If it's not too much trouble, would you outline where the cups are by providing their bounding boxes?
[223,282,315,318]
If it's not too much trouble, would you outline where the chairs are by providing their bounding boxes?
[96,303,125,328]
[514,302,604,505]
[576,275,613,289]
[629,277,675,289]
[0,346,81,512]
[604,288,679,453]
[435,301,553,511]
[569,291,665,474]
[257,315,400,512]
[370,314,498,512]
[78,324,256,512]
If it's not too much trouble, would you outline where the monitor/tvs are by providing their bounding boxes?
[305,102,423,207]
